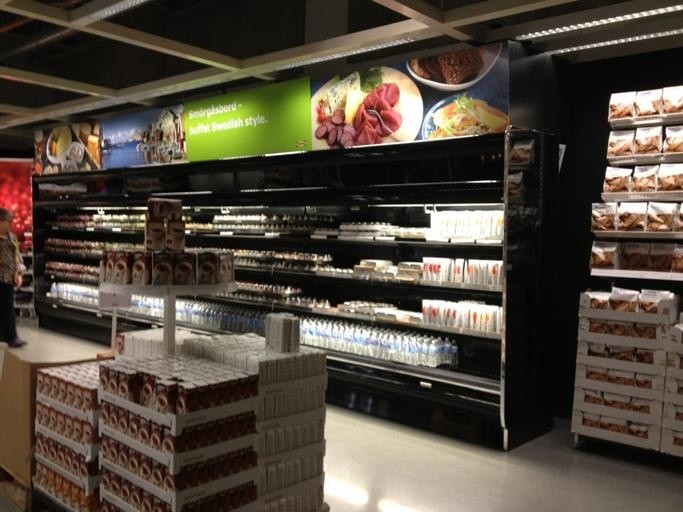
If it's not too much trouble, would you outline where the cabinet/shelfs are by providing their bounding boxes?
[98,279,332,511]
[30,128,556,455]
[570,89,682,456]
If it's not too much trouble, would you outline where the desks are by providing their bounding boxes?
[16,326,122,507]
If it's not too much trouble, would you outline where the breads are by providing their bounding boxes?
[410,49,484,84]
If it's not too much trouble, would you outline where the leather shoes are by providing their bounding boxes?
[7,337,26,347]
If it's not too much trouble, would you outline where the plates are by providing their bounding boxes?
[404,40,502,92]
[420,93,506,141]
[310,67,422,146]
[46,124,71,165]
[66,142,83,162]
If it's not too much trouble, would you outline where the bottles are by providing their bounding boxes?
[45,214,460,369]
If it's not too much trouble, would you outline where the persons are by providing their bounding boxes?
[0,209,27,349]
